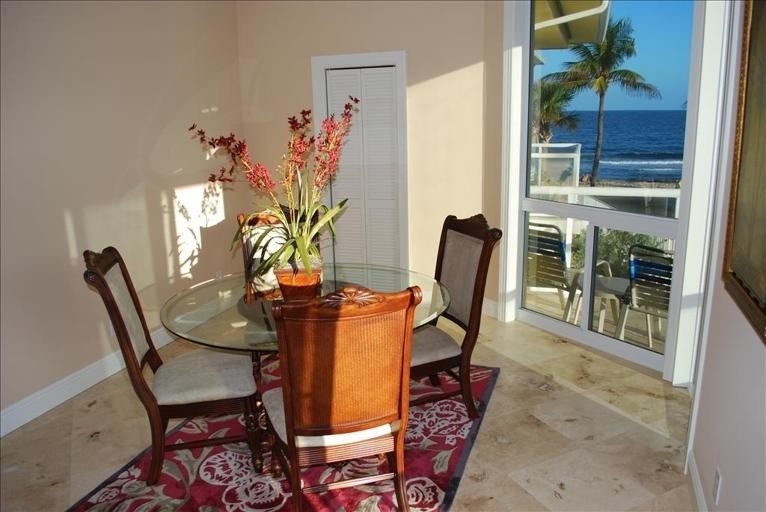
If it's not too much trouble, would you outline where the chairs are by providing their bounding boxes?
[410,214,502,419]
[236,204,321,382]
[526,221,674,351]
[262,285,422,510]
[82,245,262,487]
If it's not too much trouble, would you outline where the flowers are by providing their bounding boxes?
[186,91,363,295]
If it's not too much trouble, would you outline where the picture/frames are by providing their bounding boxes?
[722,0,766,345]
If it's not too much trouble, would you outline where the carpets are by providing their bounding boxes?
[68,364,500,512]
